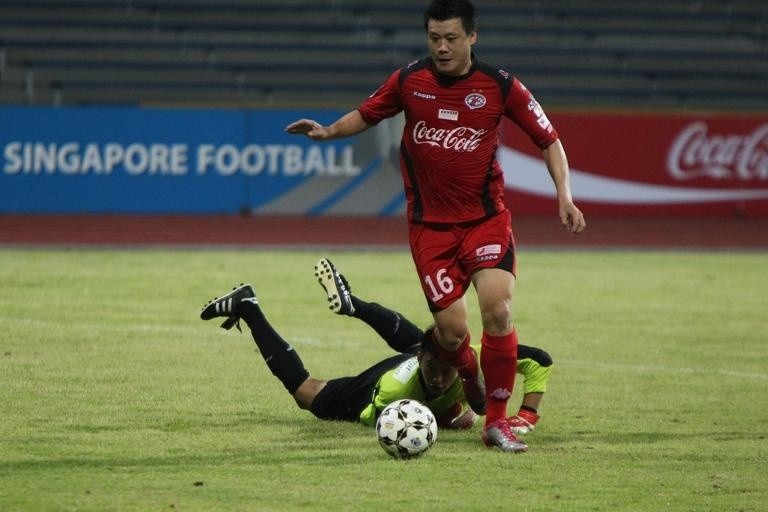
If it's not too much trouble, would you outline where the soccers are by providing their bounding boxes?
[375,398,438,461]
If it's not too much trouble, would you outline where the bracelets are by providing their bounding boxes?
[520,405,537,414]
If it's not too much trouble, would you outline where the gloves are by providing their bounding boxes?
[504,408,541,433]
[435,406,476,430]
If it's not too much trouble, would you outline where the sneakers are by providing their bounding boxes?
[313,257,352,316]
[457,348,487,416]
[200,284,258,320]
[481,419,529,452]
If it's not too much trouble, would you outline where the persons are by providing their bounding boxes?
[284,0,586,453]
[200,257,553,435]
[245,288,249,290]
